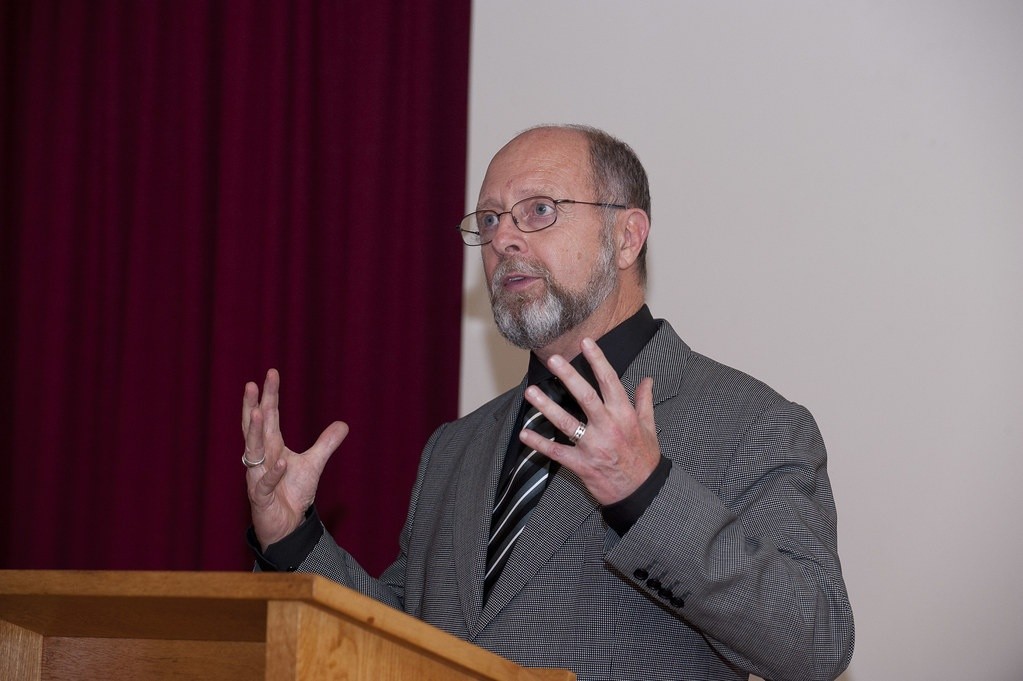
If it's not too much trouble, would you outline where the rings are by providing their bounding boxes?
[569,422,587,443]
[242,452,265,468]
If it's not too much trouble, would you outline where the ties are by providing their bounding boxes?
[483,378,572,613]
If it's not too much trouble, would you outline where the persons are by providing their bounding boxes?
[241,128,855,681]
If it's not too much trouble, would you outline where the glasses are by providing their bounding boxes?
[456,196,630,246]
